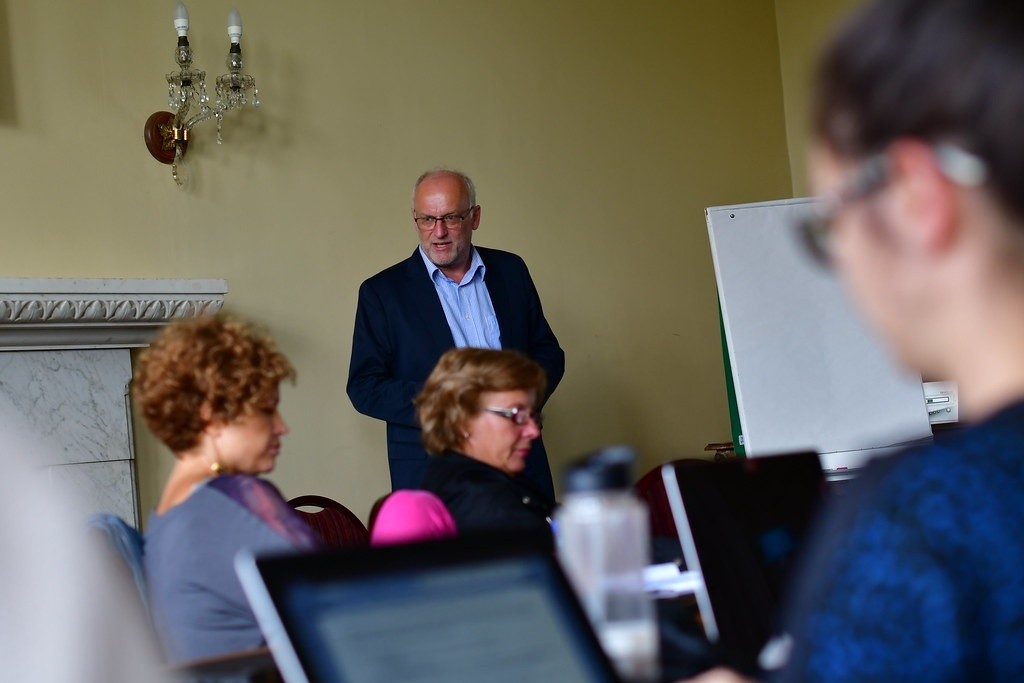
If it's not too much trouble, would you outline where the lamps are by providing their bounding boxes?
[143,0,261,191]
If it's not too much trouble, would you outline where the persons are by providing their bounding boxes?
[346,168,567,491]
[668,0,1024,683]
[128,307,454,683]
[413,347,553,547]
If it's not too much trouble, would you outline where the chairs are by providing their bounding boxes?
[287,495,368,561]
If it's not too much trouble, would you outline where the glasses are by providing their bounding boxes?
[477,404,541,427]
[414,206,475,229]
[794,144,987,265]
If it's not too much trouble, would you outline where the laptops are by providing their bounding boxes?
[234,513,628,683]
[658,451,826,641]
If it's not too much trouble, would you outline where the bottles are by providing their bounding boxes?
[550,445,661,683]
